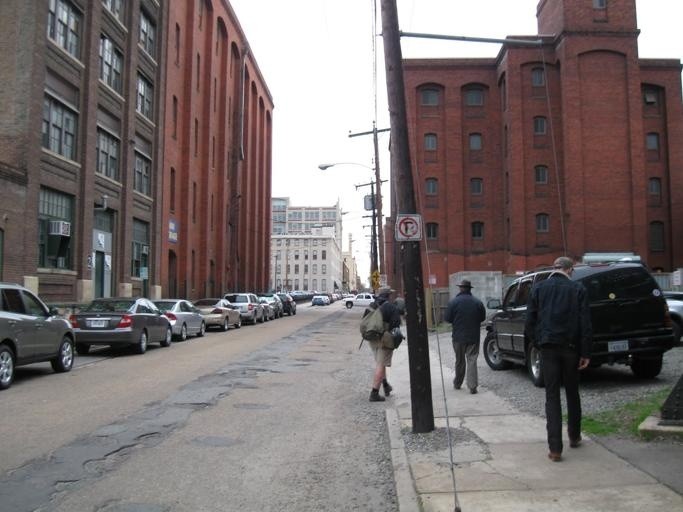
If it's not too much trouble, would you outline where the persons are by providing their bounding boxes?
[357,286,403,401]
[524,257,595,462]
[445,279,486,393]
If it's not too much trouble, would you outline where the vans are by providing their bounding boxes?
[221,292,264,325]
[482,262,675,389]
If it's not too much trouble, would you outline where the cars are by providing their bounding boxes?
[191,299,241,332]
[257,289,377,321]
[660,286,682,346]
[147,298,206,342]
[67,294,172,355]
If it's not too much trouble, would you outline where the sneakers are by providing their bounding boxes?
[454,384,460,389]
[549,453,561,461]
[369,392,385,401]
[383,384,392,396]
[470,387,477,394]
[570,436,582,447]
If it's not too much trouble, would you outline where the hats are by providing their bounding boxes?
[553,257,576,273]
[456,280,474,288]
[374,285,396,298]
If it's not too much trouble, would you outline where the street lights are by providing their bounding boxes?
[319,158,384,315]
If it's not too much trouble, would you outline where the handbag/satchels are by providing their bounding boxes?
[381,331,402,349]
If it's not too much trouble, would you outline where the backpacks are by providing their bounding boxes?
[360,301,389,340]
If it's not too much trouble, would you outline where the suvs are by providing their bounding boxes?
[0,283,74,389]
